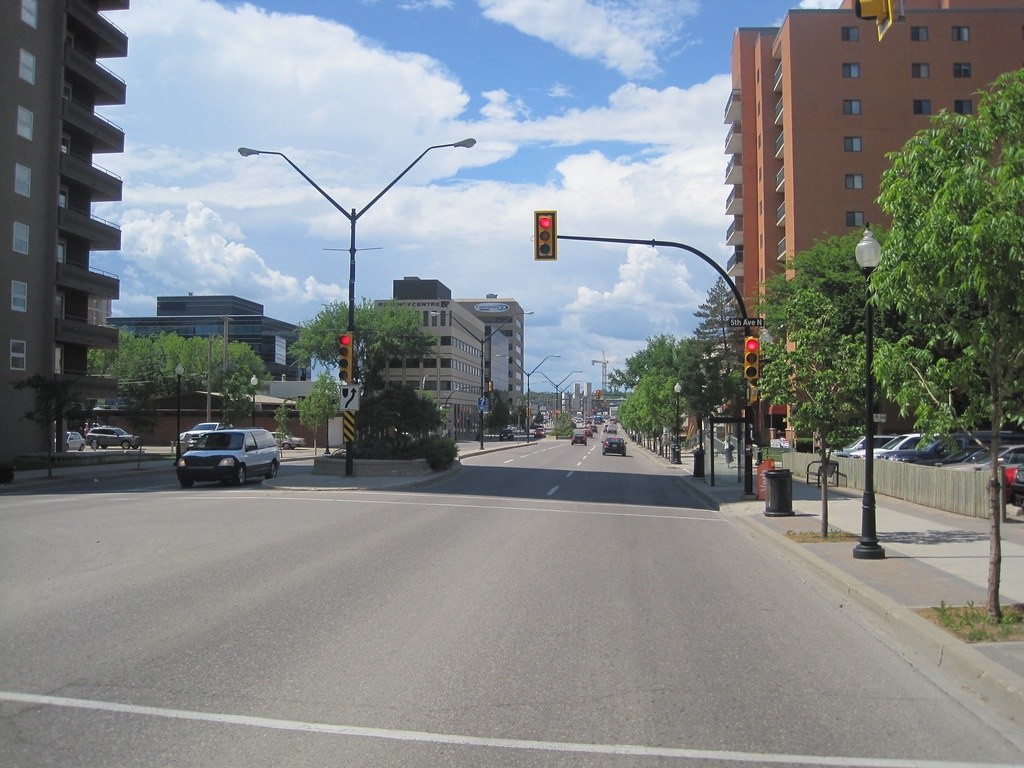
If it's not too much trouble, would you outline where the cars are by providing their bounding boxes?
[571,416,618,446]
[176,428,282,488]
[54,431,86,452]
[534,428,546,438]
[270,432,306,449]
[499,429,514,441]
[601,437,628,456]
[825,430,1024,510]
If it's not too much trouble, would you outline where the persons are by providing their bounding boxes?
[723,435,733,469]
[80,422,101,439]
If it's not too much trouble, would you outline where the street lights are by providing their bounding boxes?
[250,374,259,426]
[531,370,589,440]
[175,362,185,465]
[238,137,476,479]
[430,311,535,450]
[674,382,683,464]
[496,354,562,443]
[853,221,886,560]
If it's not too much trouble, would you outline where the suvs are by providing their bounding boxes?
[86,427,141,449]
[180,422,234,448]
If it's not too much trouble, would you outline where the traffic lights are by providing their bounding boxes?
[534,210,558,261]
[488,381,493,392]
[596,390,600,399]
[745,337,759,379]
[338,334,352,382]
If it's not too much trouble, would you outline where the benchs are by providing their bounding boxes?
[806,459,838,487]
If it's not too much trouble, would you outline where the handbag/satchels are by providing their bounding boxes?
[729,444,735,451]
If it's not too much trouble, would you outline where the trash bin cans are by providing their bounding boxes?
[692,450,705,477]
[763,469,796,517]
[670,444,681,463]
